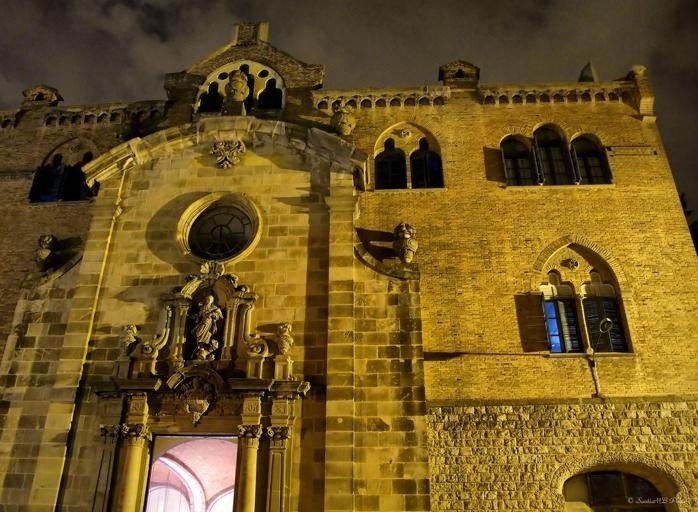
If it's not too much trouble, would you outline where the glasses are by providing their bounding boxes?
[594,318,615,352]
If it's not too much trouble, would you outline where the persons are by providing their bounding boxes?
[187,295,223,359]
